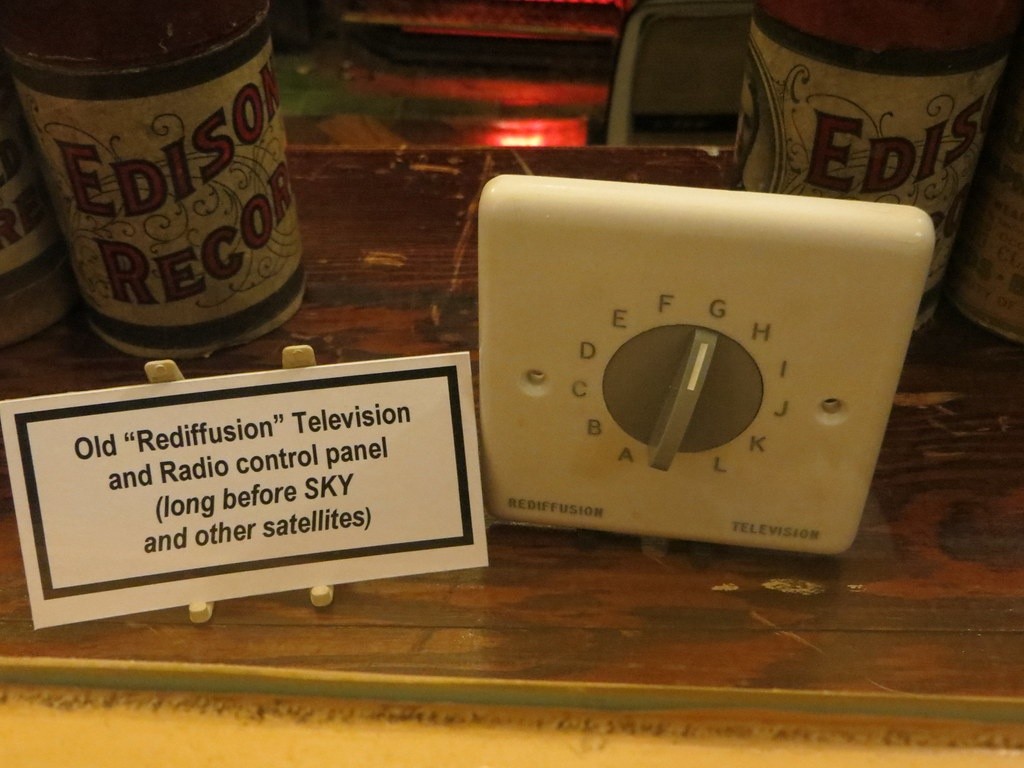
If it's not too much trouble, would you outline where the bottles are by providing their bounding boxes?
[0,0,307,360]
[0,90,82,348]
[949,78,1023,356]
[727,0,1024,360]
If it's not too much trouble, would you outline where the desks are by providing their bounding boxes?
[0,149,1024,768]
